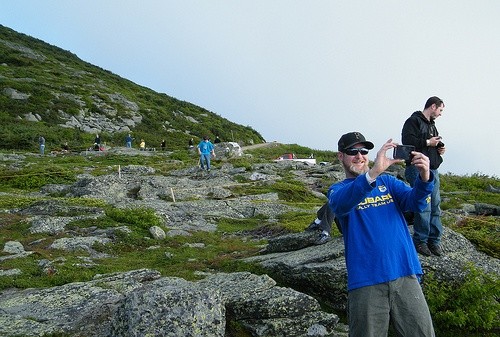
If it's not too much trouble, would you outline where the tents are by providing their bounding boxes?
[278,153,297,160]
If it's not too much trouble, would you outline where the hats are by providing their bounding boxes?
[337,132,374,150]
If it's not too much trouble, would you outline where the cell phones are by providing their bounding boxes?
[393,145,415,160]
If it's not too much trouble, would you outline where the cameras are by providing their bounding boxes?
[437,141,444,149]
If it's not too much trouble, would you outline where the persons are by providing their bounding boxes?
[94,134,100,151]
[198,135,221,172]
[62,141,69,152]
[138,137,146,152]
[125,133,135,148]
[327,131,436,336]
[38,134,46,156]
[160,139,166,151]
[401,96,447,259]
[305,173,415,245]
[188,137,194,150]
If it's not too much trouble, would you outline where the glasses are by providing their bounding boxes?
[344,148,369,156]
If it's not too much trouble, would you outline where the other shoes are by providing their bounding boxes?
[411,238,430,256]
[429,242,443,256]
[314,233,332,244]
[305,219,321,231]
[207,168,210,171]
